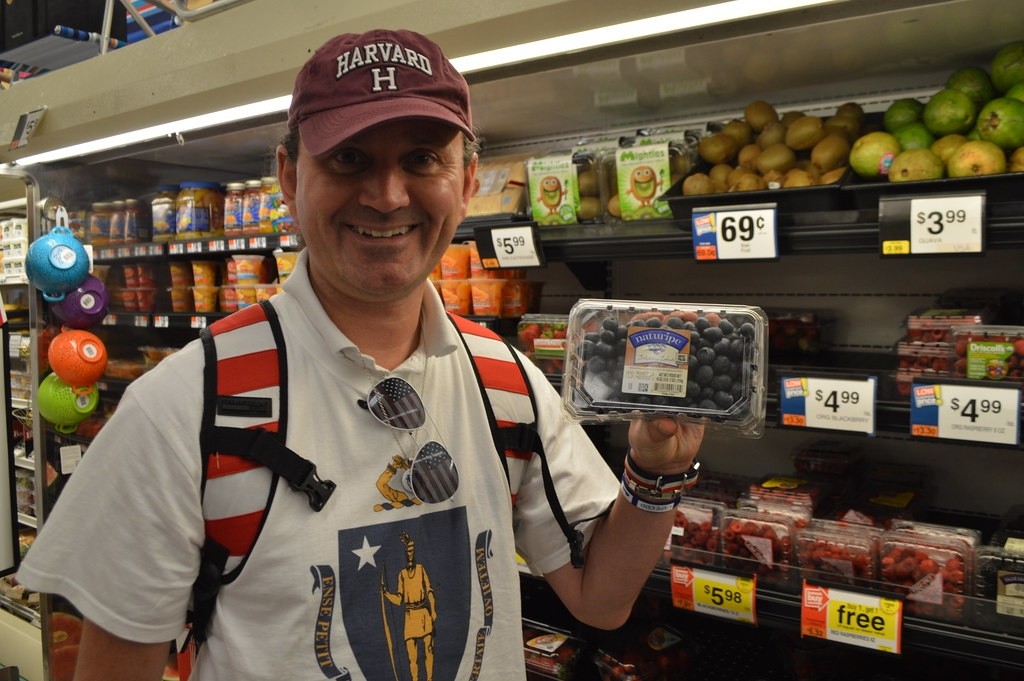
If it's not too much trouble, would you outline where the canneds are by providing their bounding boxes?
[90,174,283,248]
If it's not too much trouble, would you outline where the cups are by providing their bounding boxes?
[427,239,544,318]
[87,248,297,315]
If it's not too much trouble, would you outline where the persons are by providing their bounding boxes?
[16,29,705,680]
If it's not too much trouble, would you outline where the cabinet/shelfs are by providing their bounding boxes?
[0,5,1024,680]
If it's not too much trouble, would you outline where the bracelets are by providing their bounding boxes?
[621,447,700,511]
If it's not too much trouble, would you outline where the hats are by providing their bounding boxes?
[286,27,476,155]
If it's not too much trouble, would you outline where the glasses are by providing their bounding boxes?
[356,375,459,502]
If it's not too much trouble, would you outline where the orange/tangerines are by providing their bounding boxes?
[849,45,1024,183]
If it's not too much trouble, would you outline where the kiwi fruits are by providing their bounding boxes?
[533,99,863,223]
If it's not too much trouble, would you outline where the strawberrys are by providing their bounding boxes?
[516,312,568,375]
